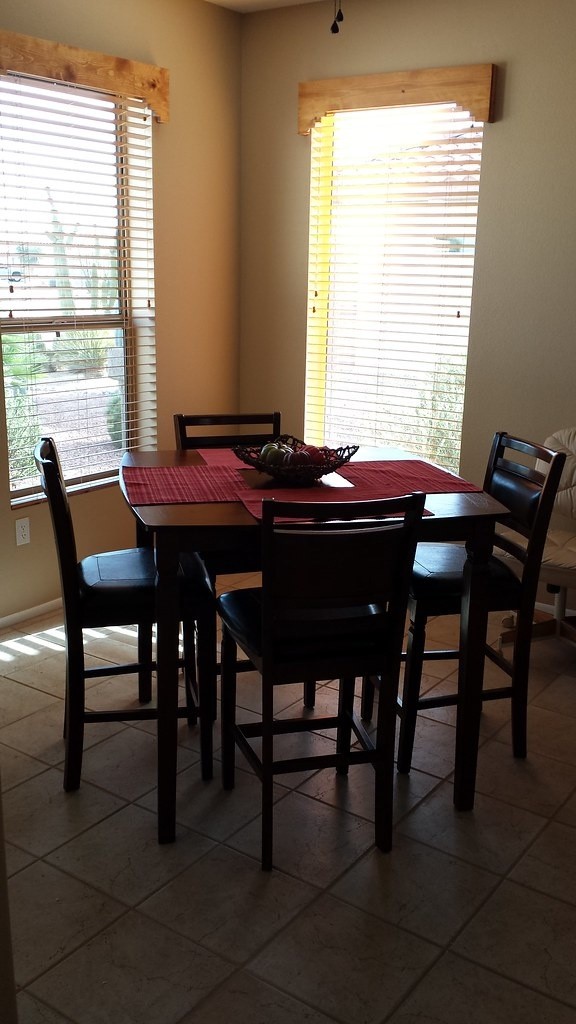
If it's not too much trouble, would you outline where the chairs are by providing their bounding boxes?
[492,428,576,646]
[360,431,565,775]
[32,436,215,796]
[215,491,427,874]
[173,411,316,722]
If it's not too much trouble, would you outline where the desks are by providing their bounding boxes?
[117,451,514,844]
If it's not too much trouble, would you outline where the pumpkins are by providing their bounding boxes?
[261,441,326,466]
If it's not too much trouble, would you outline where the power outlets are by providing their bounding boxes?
[16,517,30,546]
